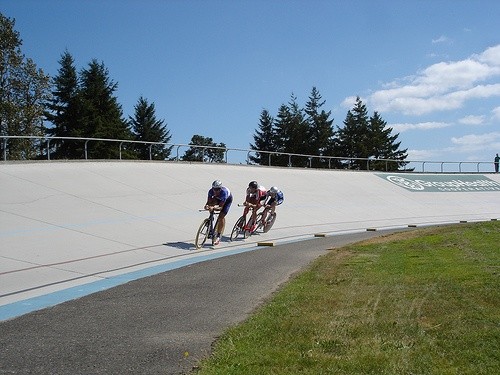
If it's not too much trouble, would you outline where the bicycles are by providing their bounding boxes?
[230,204,257,242]
[251,204,277,233]
[195,206,226,249]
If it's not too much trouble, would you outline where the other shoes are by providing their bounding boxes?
[213,234,222,245]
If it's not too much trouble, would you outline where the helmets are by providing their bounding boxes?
[212,180,222,188]
[270,186,279,194]
[248,180,258,188]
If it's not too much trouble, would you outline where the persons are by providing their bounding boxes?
[494,153,500,172]
[203,180,233,245]
[237,181,284,232]
